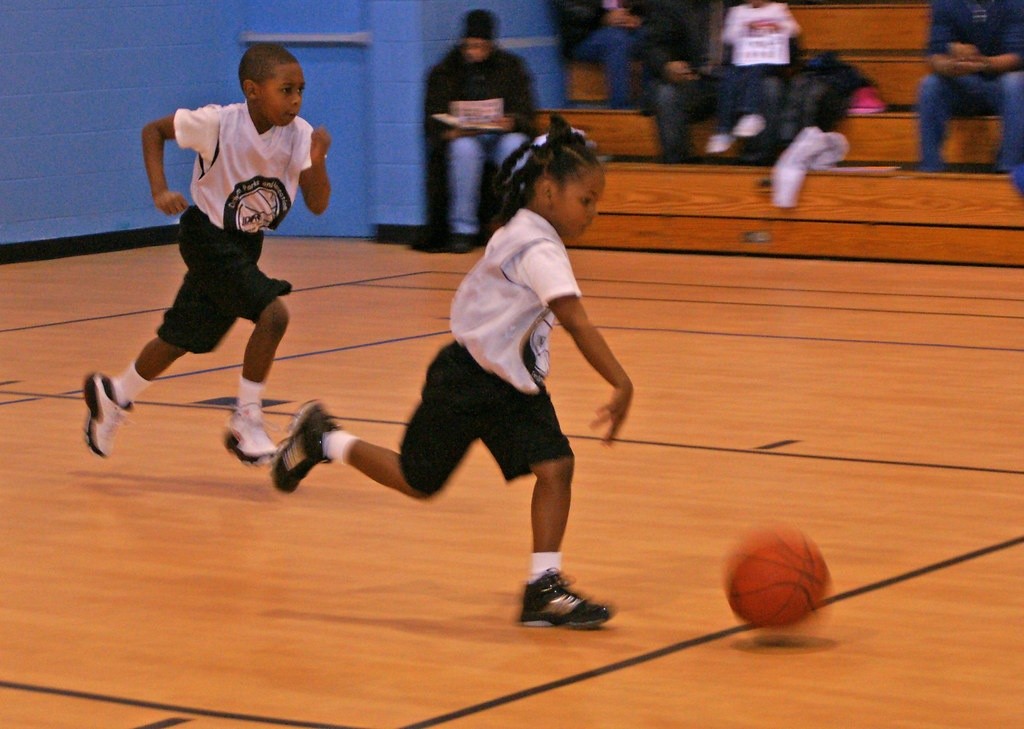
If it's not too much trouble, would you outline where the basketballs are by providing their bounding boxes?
[723,523,828,629]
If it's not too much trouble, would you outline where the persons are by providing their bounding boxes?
[557,1,804,159]
[272,113,634,627]
[84,41,333,468]
[426,9,537,251]
[917,0,1024,173]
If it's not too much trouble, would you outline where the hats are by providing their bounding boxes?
[465,11,496,43]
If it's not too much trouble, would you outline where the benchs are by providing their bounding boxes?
[530,1,1024,168]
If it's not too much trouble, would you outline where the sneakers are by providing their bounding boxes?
[517,567,612,628]
[225,402,278,466]
[84,371,134,459]
[270,398,339,495]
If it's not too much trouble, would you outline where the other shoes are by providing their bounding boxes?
[731,113,766,137]
[658,144,688,164]
[443,232,477,255]
[705,134,737,154]
